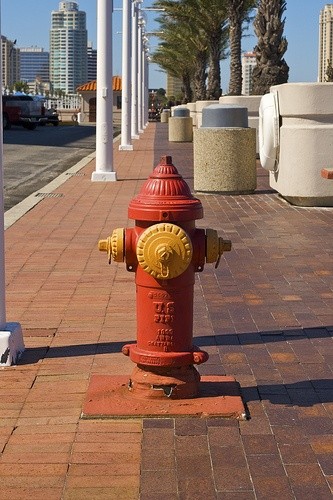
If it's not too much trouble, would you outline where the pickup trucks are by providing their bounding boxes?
[3,93,47,129]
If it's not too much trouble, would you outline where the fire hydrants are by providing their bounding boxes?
[80,156,248,419]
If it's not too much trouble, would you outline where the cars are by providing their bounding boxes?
[45,107,63,126]
